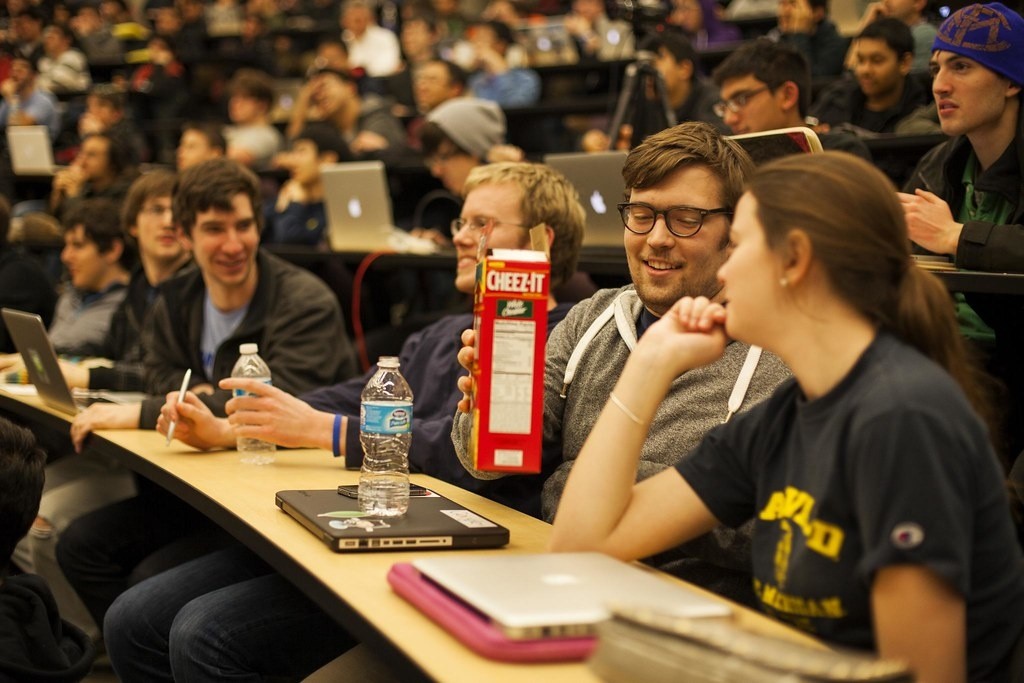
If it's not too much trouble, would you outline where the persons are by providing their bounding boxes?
[0,198,133,380]
[12,168,198,630]
[302,123,791,683]
[54,160,360,630]
[101,160,585,683]
[547,151,1024,683]
[0,0,1023,312]
[0,417,94,683]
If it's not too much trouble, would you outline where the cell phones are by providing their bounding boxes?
[337,484,426,498]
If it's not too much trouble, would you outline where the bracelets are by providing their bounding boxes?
[609,392,644,425]
[333,414,343,457]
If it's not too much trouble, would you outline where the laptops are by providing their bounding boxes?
[410,548,734,641]
[519,23,579,66]
[725,127,824,169]
[275,486,510,554]
[6,125,70,175]
[545,151,635,248]
[1,308,155,415]
[319,161,438,254]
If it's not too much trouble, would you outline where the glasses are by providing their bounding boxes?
[616,201,730,238]
[714,83,777,116]
[452,219,538,237]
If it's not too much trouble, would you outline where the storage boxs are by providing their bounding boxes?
[467,246,553,474]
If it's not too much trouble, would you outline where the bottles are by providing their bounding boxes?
[229,342,279,466]
[358,356,413,517]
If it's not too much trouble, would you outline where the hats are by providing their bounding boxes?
[426,98,506,162]
[931,3,1024,87]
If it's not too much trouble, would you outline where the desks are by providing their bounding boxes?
[0,0,1024,683]
